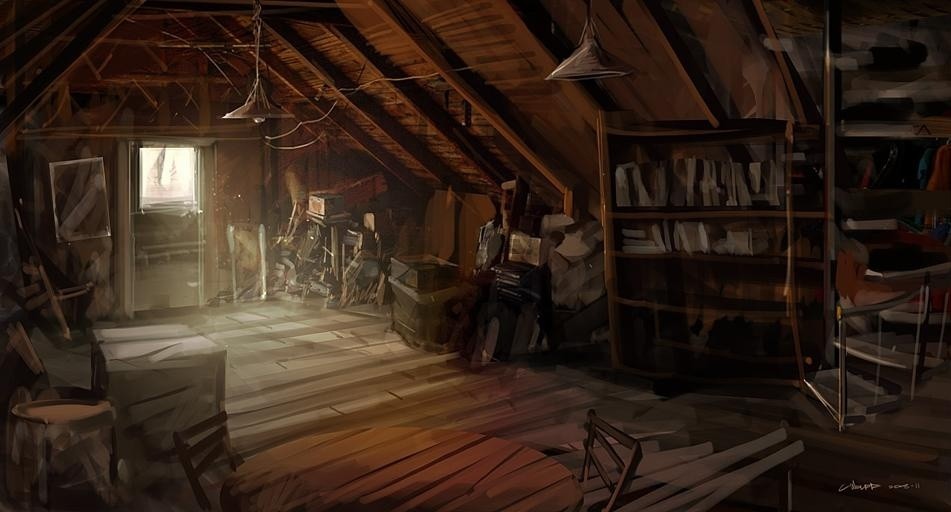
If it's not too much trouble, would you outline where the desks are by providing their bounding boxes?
[220,418,588,512]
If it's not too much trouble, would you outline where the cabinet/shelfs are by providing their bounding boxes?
[599,119,829,404]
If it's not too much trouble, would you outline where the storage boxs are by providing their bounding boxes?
[87,325,227,461]
[309,193,344,217]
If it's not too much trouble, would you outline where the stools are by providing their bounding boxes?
[4,393,125,509]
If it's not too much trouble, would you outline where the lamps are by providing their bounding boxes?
[220,0,297,123]
[542,1,638,83]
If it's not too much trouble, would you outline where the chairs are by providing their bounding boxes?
[579,408,647,512]
